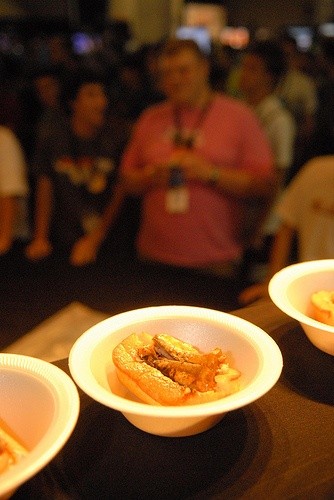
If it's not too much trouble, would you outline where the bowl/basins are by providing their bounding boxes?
[268,259,333,356]
[68,304,283,438]
[1,352,80,500]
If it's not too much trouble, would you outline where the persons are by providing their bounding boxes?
[239,154,334,301]
[119,38,278,286]
[0,18,166,264]
[209,25,334,194]
